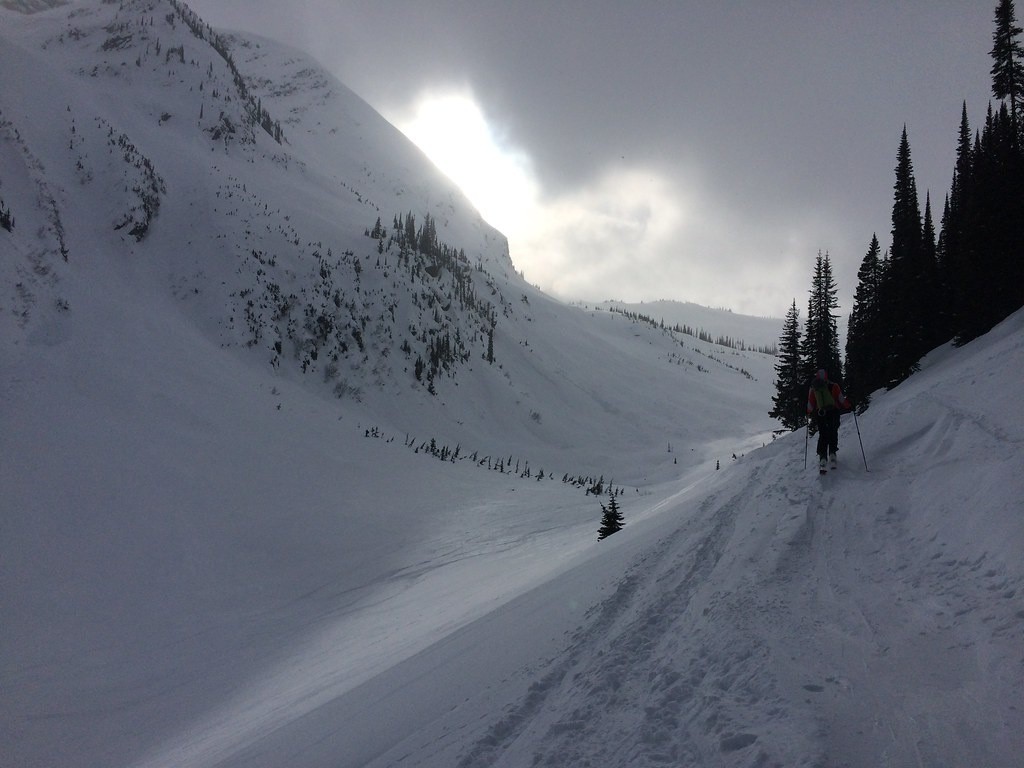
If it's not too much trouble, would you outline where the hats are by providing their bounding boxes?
[816,369,828,382]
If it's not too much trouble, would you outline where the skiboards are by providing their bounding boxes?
[818,459,838,475]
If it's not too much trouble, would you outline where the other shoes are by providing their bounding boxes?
[830,454,837,470]
[820,458,828,472]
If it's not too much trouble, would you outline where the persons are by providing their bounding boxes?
[806,369,853,474]
